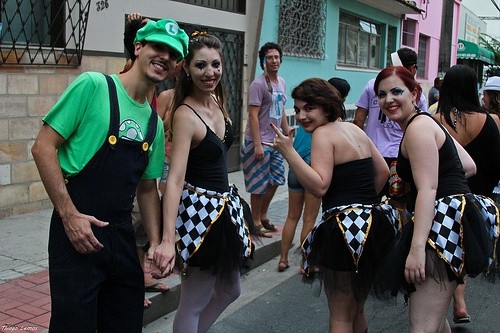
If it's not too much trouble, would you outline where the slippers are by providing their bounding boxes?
[145,281,169,293]
[453,313,471,323]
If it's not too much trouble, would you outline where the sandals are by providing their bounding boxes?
[278,260,290,271]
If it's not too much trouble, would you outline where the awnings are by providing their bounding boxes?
[457,39,495,65]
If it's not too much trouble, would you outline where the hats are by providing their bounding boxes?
[482,76,500,91]
[133,19,190,64]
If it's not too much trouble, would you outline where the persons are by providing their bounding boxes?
[240,41,293,238]
[30,18,254,333]
[354,48,426,220]
[374,66,476,333]
[260,79,389,333]
[431,66,500,279]
[278,76,351,274]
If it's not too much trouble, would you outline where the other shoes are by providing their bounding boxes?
[252,225,272,238]
[261,219,278,231]
